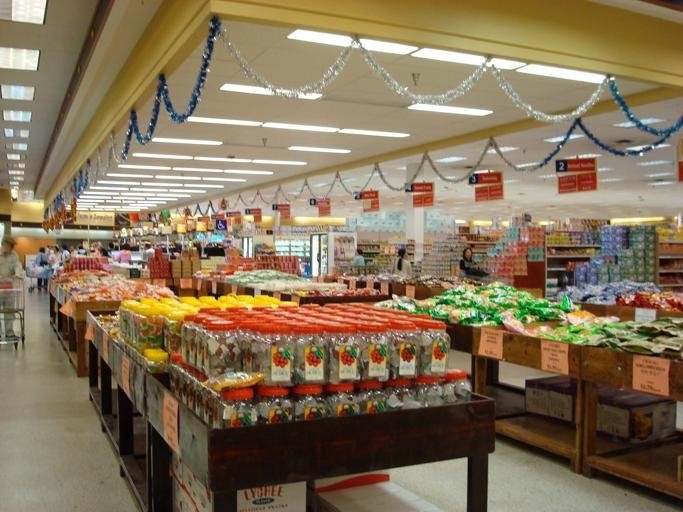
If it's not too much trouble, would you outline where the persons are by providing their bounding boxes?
[352,249,365,267]
[34,241,156,292]
[459,248,482,276]
[393,248,413,275]
[0,234,25,339]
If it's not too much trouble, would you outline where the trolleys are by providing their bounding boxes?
[0,274,26,348]
[25,267,52,292]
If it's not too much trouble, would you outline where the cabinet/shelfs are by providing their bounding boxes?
[47,231,683,512]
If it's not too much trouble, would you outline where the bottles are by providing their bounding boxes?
[118,294,471,428]
